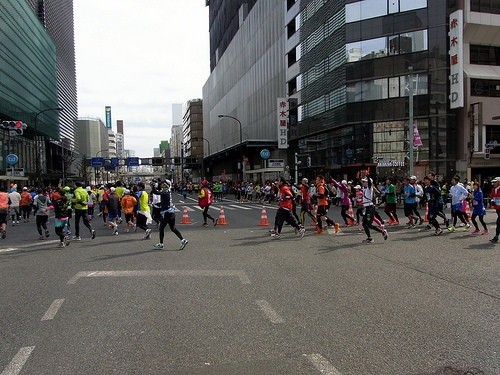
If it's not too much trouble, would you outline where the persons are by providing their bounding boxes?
[172,180,227,226]
[269,172,488,244]
[0,180,188,250]
[489,177,500,242]
[233,181,276,204]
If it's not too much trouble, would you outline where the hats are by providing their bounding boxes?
[86,186,91,191]
[353,185,362,189]
[303,178,308,183]
[110,187,116,194]
[410,176,418,181]
[164,179,171,188]
[202,180,208,186]
[491,177,500,182]
[99,185,104,189]
[341,180,347,186]
[23,187,27,190]
[362,177,374,184]
[63,186,71,192]
[10,186,17,192]
[123,189,130,195]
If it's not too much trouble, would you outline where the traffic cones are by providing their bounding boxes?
[347,205,357,225]
[310,205,320,226]
[465,201,473,220]
[179,207,191,224]
[217,206,228,225]
[423,202,430,222]
[257,208,271,226]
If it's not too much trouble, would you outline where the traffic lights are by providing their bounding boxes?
[2,120,27,135]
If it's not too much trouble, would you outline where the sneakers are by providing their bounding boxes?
[153,243,164,249]
[268,216,489,243]
[201,222,209,226]
[144,229,152,238]
[213,218,218,227]
[142,237,150,240]
[180,239,188,250]
[0,214,138,248]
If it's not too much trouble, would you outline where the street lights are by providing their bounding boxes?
[194,136,210,178]
[218,115,243,181]
[35,108,63,181]
[96,149,108,184]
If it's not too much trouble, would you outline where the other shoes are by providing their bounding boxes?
[490,237,499,242]
[155,222,160,228]
